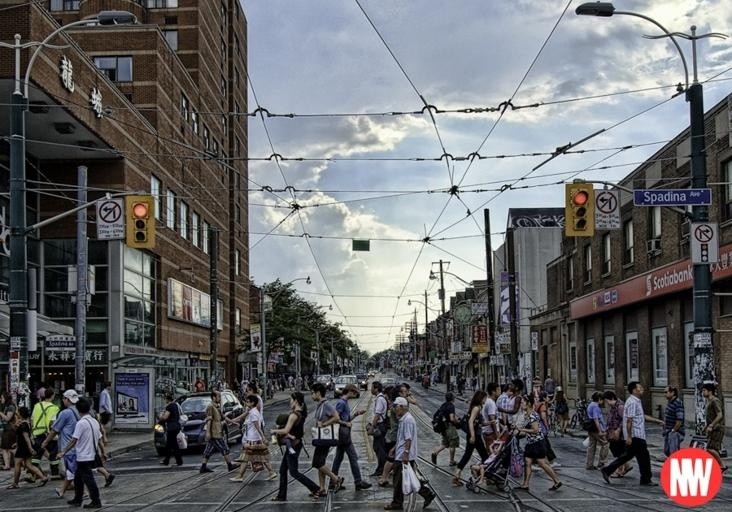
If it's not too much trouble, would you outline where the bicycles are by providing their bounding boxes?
[544,399,565,438]
[567,396,591,429]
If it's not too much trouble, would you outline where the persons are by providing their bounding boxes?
[0,372,726,512]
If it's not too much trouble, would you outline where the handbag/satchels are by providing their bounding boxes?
[242,438,270,455]
[92,452,104,469]
[310,397,341,448]
[457,401,481,435]
[509,436,524,478]
[578,436,589,448]
[382,409,397,445]
[176,430,187,450]
[400,461,422,495]
[606,429,620,440]
[580,416,599,433]
[175,401,189,425]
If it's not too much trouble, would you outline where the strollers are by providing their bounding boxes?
[690,422,713,450]
[465,425,525,493]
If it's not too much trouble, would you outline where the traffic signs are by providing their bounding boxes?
[634,186,713,207]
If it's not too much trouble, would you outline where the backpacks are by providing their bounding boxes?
[431,401,454,434]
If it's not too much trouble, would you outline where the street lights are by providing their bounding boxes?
[405,299,442,382]
[10,8,139,409]
[429,271,497,392]
[262,273,313,403]
[295,302,335,391]
[575,1,716,443]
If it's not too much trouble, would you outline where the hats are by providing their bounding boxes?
[392,396,409,407]
[62,388,80,405]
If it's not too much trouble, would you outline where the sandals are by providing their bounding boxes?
[448,460,458,466]
[431,451,437,465]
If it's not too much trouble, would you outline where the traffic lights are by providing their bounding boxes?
[701,244,709,262]
[125,196,154,248]
[566,184,595,236]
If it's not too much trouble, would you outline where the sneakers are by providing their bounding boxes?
[639,480,659,488]
[531,462,562,472]
[583,464,635,484]
[197,463,279,483]
[512,485,529,490]
[384,503,405,511]
[159,458,183,466]
[66,496,103,508]
[270,495,287,502]
[104,472,115,487]
[451,475,464,486]
[422,490,436,509]
[548,480,562,492]
[307,478,374,500]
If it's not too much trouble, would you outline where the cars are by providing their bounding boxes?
[312,369,399,400]
[154,389,247,456]
